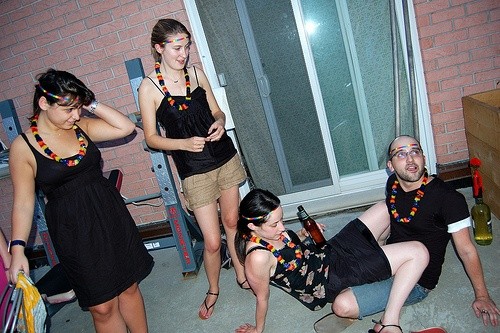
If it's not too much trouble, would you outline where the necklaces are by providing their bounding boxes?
[154,61,190,111]
[390,167,428,223]
[233,188,430,333]
[243,233,302,270]
[30,111,88,167]
[162,71,184,83]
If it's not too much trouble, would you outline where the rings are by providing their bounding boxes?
[481,309,488,314]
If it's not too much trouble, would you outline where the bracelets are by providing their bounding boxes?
[88,99,100,113]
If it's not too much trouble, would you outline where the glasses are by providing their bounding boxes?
[391,150,421,160]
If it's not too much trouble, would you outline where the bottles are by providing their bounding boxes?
[296,206,326,246]
[469,186,493,246]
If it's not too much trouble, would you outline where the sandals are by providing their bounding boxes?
[236,278,256,296]
[199,288,219,320]
[368,319,404,333]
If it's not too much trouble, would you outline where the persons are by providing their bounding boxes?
[137,19,252,321]
[6,67,155,333]
[0,228,12,333]
[331,134,500,328]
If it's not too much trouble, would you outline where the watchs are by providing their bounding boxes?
[7,239,26,255]
